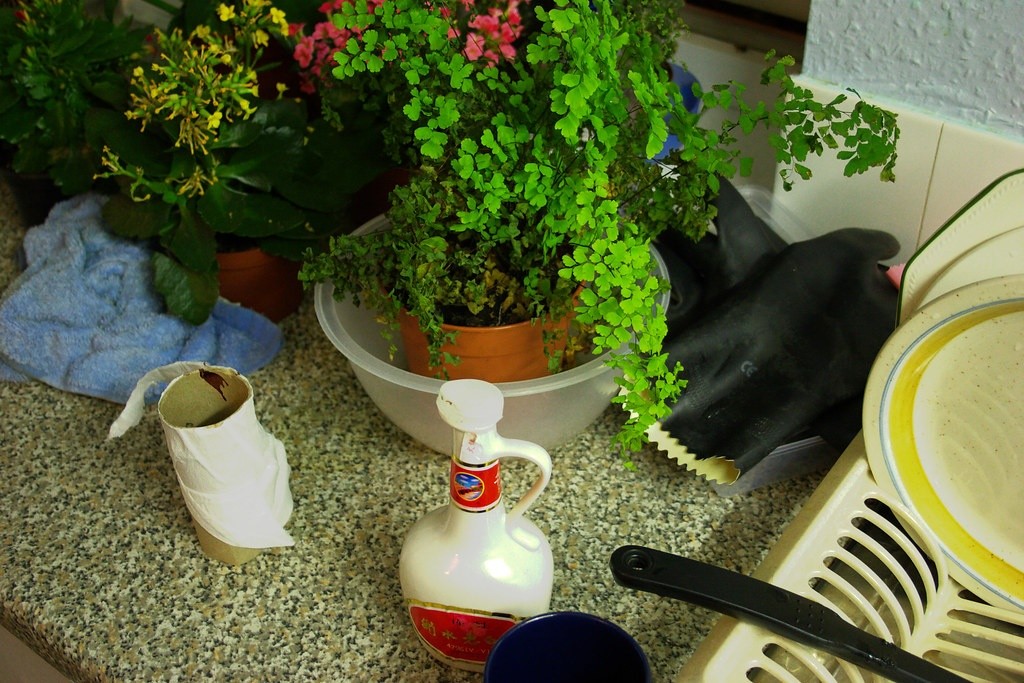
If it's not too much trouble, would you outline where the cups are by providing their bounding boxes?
[483,611,652,683]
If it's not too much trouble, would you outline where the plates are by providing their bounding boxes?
[894,168,1024,328]
[861,274,1024,617]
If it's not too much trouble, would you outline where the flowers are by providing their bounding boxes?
[90,0,319,325]
[286,0,903,477]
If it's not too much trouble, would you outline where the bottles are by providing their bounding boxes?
[398,378,554,672]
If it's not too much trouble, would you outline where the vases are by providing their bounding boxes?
[311,211,670,461]
[215,243,304,323]
[380,273,590,381]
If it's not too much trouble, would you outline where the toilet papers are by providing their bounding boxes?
[101,360,295,564]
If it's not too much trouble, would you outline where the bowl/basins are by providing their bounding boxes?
[313,206,672,458]
